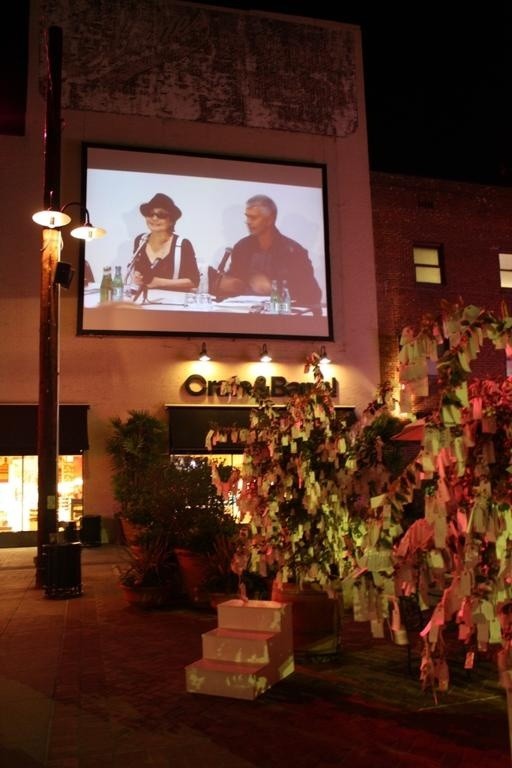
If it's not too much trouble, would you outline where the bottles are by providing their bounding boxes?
[101,267,113,304]
[112,267,123,303]
[270,281,280,314]
[281,281,291,314]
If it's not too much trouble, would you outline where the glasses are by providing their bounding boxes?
[146,208,167,219]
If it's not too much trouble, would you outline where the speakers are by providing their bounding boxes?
[55,261,72,287]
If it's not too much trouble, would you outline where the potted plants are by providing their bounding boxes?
[224,373,348,657]
[169,456,220,608]
[209,575,228,611]
[106,409,168,559]
[117,543,168,608]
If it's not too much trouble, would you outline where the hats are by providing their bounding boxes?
[139,192,182,219]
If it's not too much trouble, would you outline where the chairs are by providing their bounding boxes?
[399,591,471,683]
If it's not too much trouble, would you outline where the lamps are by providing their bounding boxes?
[198,340,210,362]
[318,344,332,369]
[258,343,274,363]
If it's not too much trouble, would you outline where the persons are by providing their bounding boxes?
[220,195,321,299]
[128,193,200,292]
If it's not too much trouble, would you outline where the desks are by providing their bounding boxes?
[42,540,82,597]
